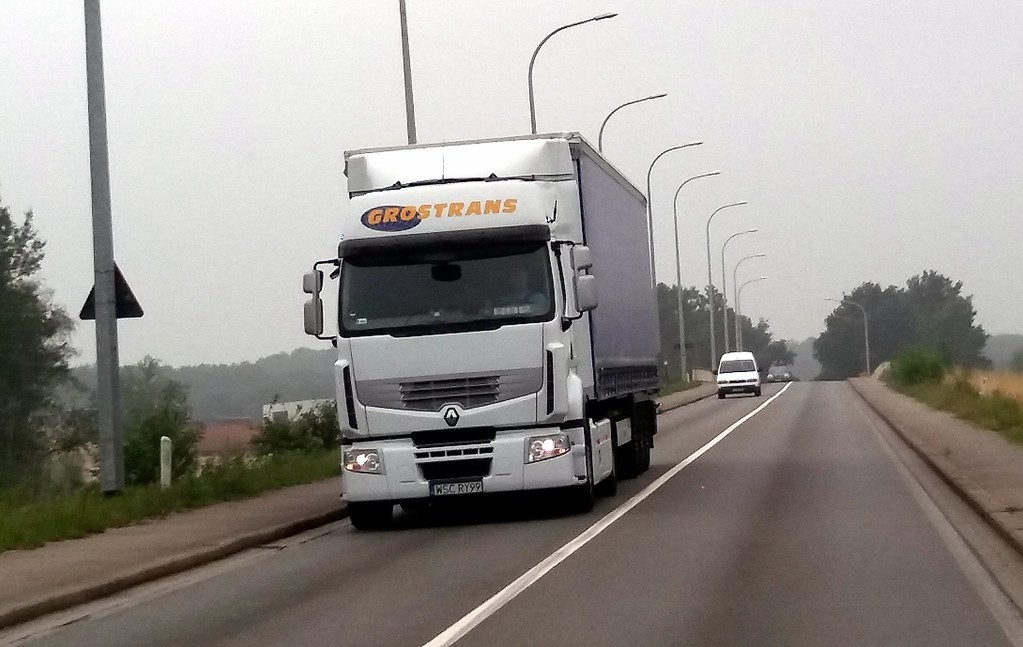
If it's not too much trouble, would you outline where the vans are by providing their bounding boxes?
[716,351,762,401]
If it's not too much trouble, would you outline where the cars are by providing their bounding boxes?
[769,365,792,383]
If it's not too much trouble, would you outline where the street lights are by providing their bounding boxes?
[706,201,747,374]
[733,253,765,352]
[597,93,667,155]
[673,171,720,382]
[826,297,872,378]
[529,13,616,136]
[721,229,756,354]
[647,143,703,315]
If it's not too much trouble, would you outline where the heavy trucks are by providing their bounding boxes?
[302,132,663,530]
